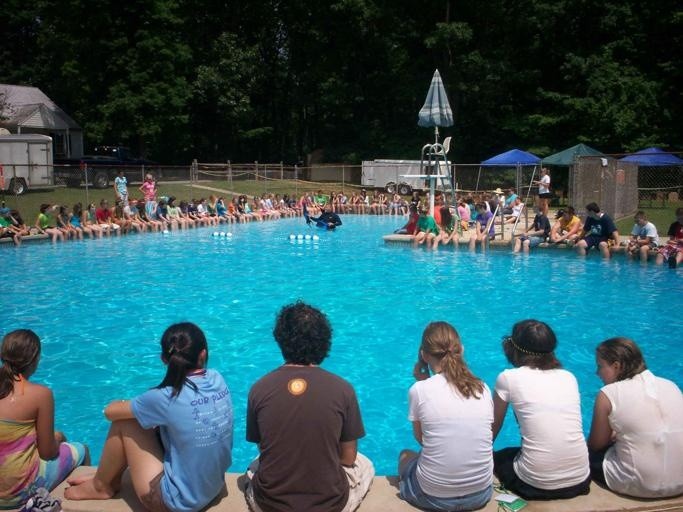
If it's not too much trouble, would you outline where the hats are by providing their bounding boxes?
[493,188,505,193]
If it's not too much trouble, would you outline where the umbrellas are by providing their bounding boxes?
[618,147,683,166]
[481,148,541,195]
[417,69,454,152]
[541,144,617,167]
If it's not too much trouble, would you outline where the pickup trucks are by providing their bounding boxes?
[54,144,160,189]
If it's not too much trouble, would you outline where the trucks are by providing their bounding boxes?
[0,128,54,195]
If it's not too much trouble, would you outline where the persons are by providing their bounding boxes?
[310,203,342,229]
[491,319,591,501]
[513,205,550,252]
[35,199,122,241]
[1,202,30,245]
[0,329,91,510]
[398,322,494,512]
[586,337,683,498]
[656,208,682,268]
[533,168,551,216]
[114,170,129,200]
[624,211,660,263]
[139,173,158,202]
[64,322,234,512]
[329,188,409,216]
[244,299,375,512]
[397,187,524,251]
[575,203,620,257]
[121,193,230,233]
[228,189,327,223]
